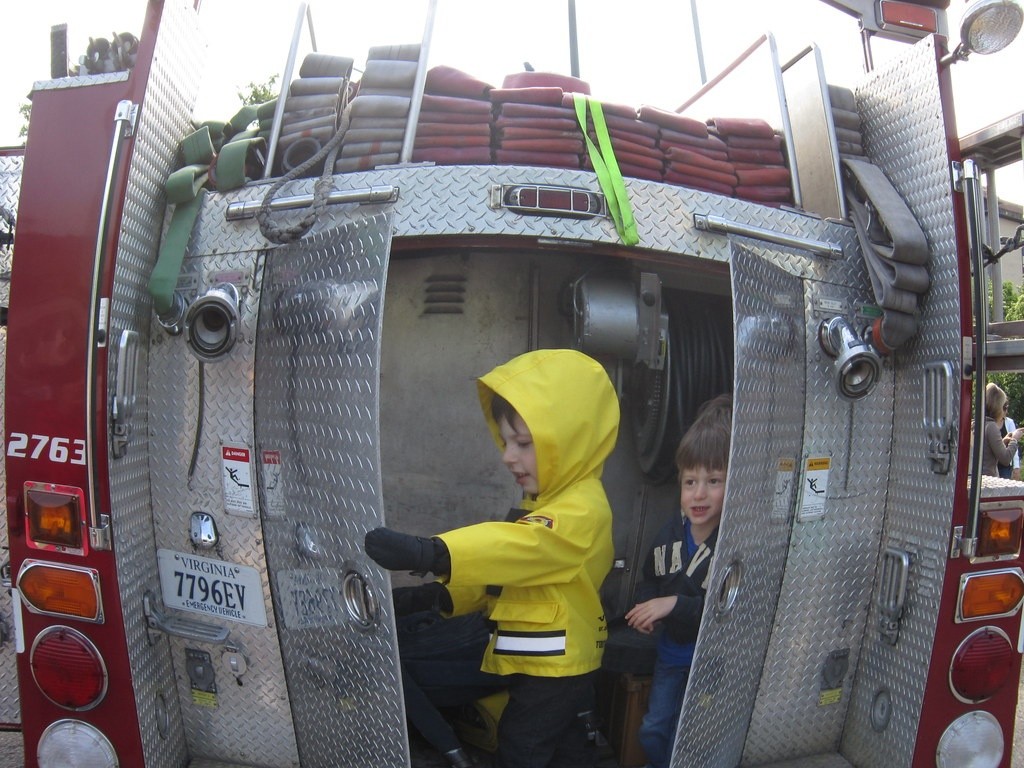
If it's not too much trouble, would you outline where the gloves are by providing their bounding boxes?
[392,582,452,617]
[363,527,451,579]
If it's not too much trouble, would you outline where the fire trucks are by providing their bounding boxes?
[3,0,1023,767]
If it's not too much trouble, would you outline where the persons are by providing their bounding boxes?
[968,383,1024,481]
[626,396,734,768]
[365,348,621,768]
